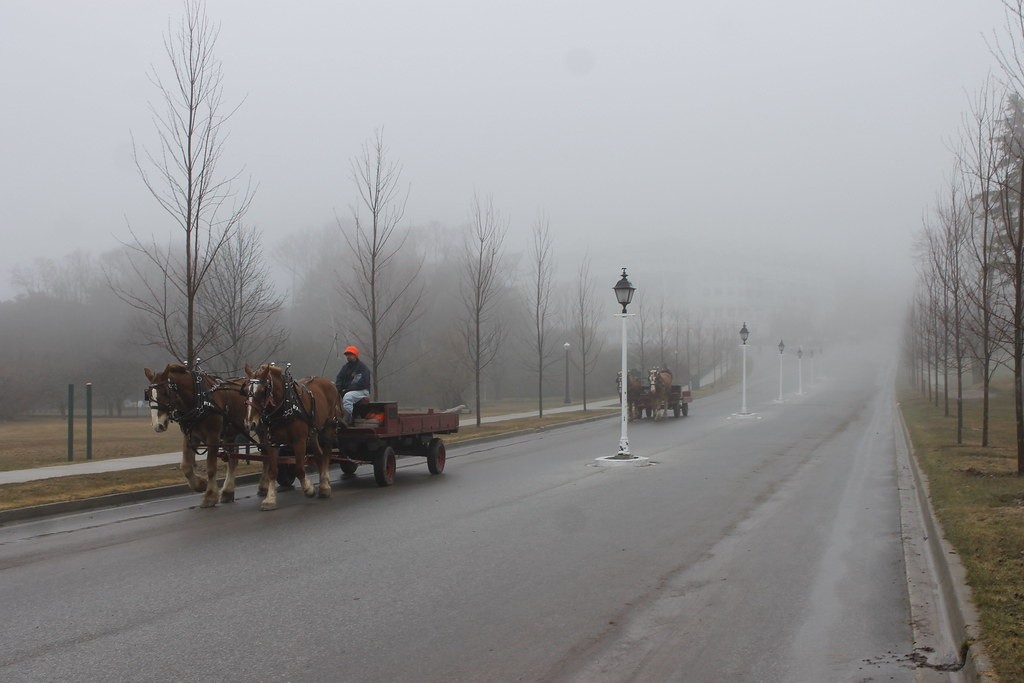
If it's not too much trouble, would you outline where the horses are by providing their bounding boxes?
[616,367,673,423]
[144,362,346,510]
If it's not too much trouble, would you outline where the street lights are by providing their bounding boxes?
[611,267,634,455]
[738,321,750,414]
[778,340,784,400]
[562,342,573,403]
[796,347,802,395]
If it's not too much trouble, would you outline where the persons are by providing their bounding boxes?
[335,346,371,424]
[661,364,674,380]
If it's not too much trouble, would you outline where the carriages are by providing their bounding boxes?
[614,366,694,423]
[142,362,461,512]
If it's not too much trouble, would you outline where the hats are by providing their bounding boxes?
[344,346,359,358]
